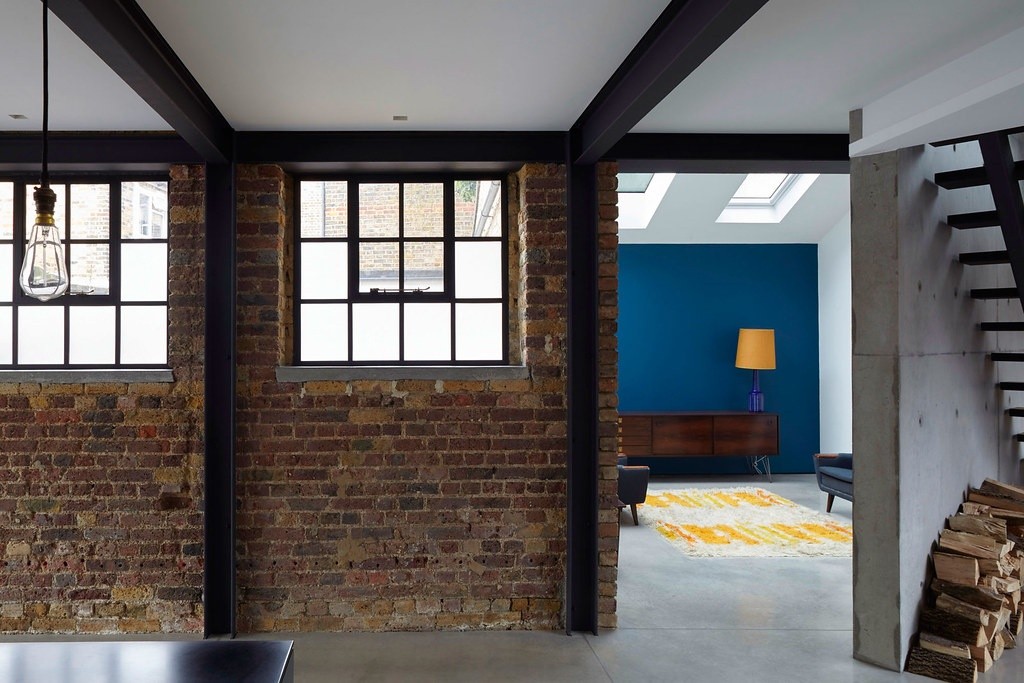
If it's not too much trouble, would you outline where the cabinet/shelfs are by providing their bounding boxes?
[618,412,781,483]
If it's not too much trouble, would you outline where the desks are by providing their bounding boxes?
[0,640,296,683]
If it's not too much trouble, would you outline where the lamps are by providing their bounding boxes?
[18,3,71,305]
[734,328,778,413]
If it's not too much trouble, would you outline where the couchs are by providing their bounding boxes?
[812,453,852,512]
[617,453,650,526]
